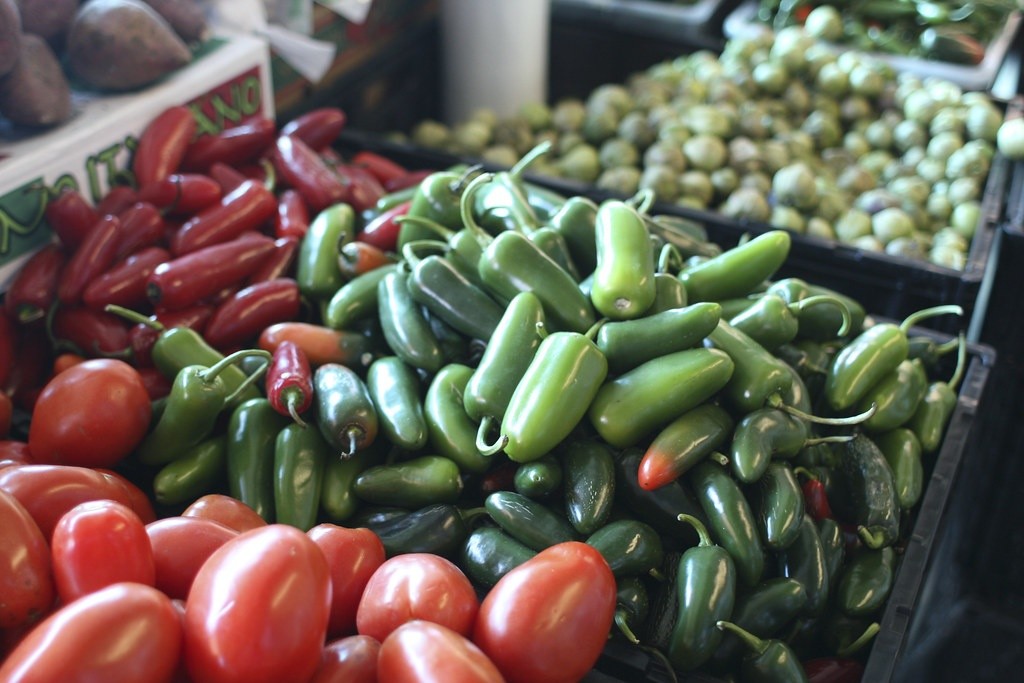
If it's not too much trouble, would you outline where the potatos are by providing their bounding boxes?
[1,0,213,131]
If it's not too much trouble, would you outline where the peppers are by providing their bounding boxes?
[753,0,1024,65]
[0,109,970,683]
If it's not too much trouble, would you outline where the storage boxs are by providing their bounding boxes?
[0,0,1024,683]
